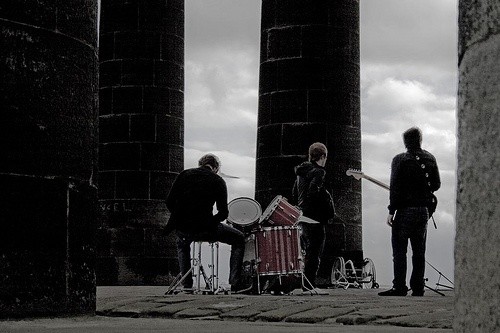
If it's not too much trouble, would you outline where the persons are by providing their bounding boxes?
[292,142,335,292]
[163,154,252,294]
[378,128,441,297]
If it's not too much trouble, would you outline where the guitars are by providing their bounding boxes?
[345,167,436,221]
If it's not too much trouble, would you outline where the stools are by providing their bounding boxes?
[169,237,215,295]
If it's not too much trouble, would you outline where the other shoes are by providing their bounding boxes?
[378,287,406,297]
[302,287,329,295]
[184,282,192,291]
[231,281,252,293]
[411,291,425,296]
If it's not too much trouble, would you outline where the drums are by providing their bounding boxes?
[251,225,305,275]
[258,195,303,227]
[226,197,263,234]
